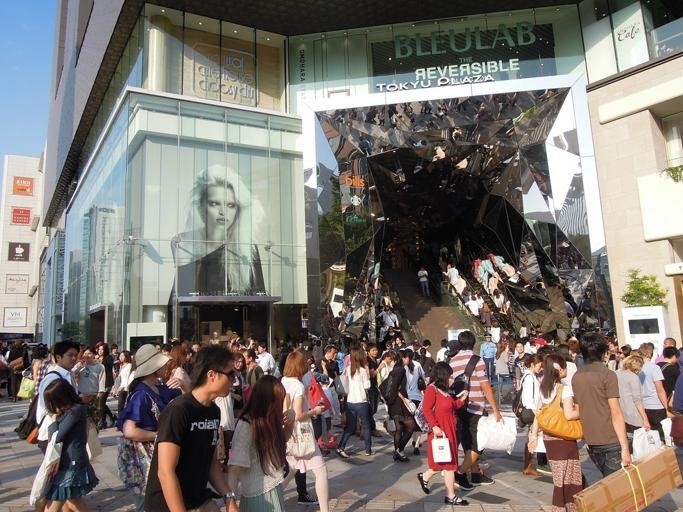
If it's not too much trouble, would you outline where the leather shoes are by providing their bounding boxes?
[418,473,430,494]
[414,448,420,455]
[412,441,422,447]
[445,496,468,505]
[393,450,409,462]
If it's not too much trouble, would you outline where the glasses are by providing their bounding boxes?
[213,370,235,376]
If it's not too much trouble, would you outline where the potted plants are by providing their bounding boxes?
[618,265,670,308]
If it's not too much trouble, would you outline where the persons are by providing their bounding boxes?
[416,267,433,299]
[377,280,401,329]
[316,89,565,197]
[436,243,494,327]
[472,230,614,327]
[315,262,377,344]
[2,322,681,509]
[166,162,269,298]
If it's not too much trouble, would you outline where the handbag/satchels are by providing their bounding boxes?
[632,428,660,459]
[537,403,584,440]
[30,431,63,506]
[447,374,470,414]
[432,432,452,462]
[477,415,517,456]
[512,390,526,417]
[17,377,35,398]
[379,373,402,401]
[306,383,331,414]
[418,377,426,390]
[118,436,154,496]
[660,418,675,448]
[286,421,316,459]
[415,391,435,433]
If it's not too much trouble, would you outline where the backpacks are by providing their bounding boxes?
[18,371,63,444]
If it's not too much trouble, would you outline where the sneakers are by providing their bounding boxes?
[471,472,494,487]
[297,492,318,504]
[454,470,473,491]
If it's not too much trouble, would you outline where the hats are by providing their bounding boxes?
[133,344,173,379]
[484,334,491,338]
[518,409,534,428]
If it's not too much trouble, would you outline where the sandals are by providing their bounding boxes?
[336,448,349,458]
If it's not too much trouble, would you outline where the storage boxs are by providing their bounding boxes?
[571,443,683,512]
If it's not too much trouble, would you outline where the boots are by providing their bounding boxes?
[523,443,544,477]
